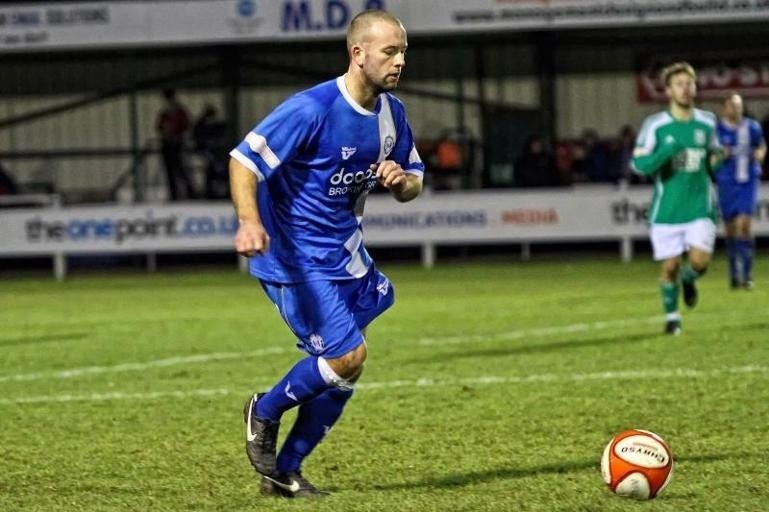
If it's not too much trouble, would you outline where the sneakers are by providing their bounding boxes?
[242,392,280,478]
[257,469,332,499]
[664,319,682,338]
[679,265,701,308]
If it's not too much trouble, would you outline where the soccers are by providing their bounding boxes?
[601,430,672,500]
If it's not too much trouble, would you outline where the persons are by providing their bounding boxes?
[156,82,228,200]
[717,89,767,289]
[429,126,652,184]
[629,61,726,334]
[230,10,424,500]
